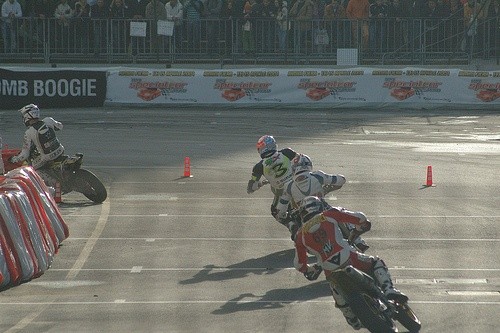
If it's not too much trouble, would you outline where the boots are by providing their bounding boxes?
[373,266,408,304]
[336,303,360,330]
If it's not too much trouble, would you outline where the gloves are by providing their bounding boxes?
[355,221,371,232]
[306,267,316,278]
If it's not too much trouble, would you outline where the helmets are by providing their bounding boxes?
[18,103,40,124]
[258,135,278,158]
[290,154,312,176]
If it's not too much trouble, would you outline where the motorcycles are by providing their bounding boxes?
[247,164,424,333]
[7,142,107,204]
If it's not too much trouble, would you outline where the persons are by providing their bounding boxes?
[0,0,500,56]
[247,135,298,241]
[274,155,369,254]
[7,103,71,195]
[293,196,408,330]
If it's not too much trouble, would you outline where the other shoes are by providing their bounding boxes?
[290,221,298,240]
[355,238,371,253]
